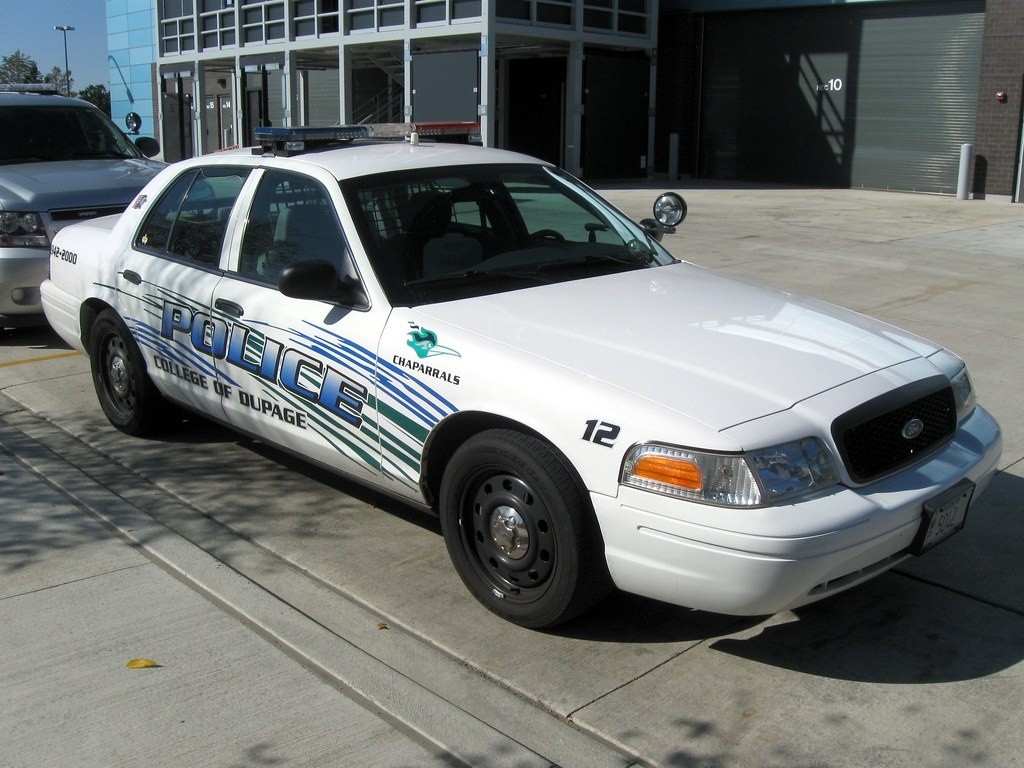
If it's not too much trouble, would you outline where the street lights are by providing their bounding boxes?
[53,25,75,97]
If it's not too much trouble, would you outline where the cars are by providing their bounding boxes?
[39,119,1004,633]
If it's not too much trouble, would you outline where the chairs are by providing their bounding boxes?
[387,189,494,278]
[256,205,344,279]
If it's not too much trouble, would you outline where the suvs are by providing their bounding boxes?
[0,82,221,333]
